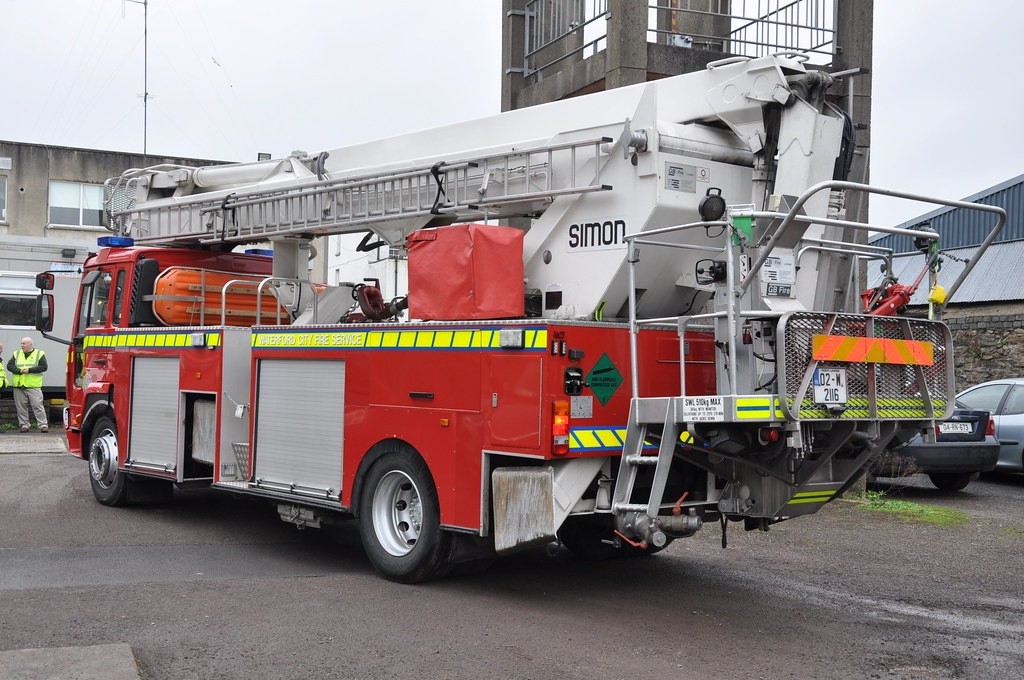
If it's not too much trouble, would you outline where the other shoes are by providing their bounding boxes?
[41,427,48,432]
[20,428,29,433]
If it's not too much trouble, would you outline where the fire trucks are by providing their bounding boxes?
[31,50,1012,588]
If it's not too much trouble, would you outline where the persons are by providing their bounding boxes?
[0,344,9,433]
[7,337,48,432]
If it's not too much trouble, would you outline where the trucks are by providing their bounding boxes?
[0,273,84,409]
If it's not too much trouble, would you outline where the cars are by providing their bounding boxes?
[954,378,1024,472]
[856,377,1000,491]
